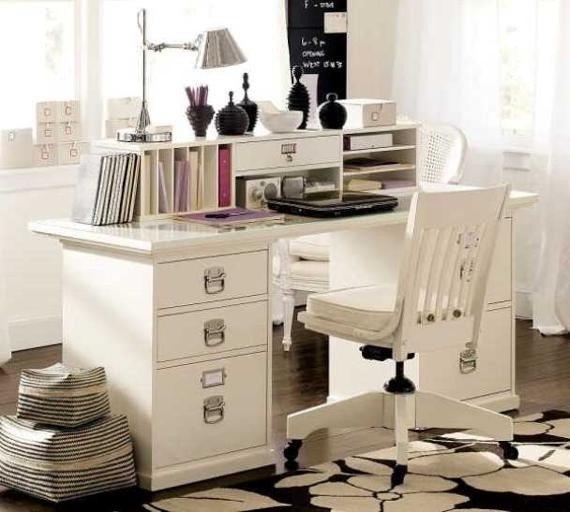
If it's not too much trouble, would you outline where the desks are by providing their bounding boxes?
[24,181,544,492]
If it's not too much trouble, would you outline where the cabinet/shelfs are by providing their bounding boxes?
[76,128,417,223]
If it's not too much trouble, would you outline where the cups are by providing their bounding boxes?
[187,105,215,136]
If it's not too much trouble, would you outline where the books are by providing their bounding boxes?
[69,144,287,228]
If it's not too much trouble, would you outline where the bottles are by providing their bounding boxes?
[216,63,347,135]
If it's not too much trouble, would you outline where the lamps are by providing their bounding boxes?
[114,6,247,144]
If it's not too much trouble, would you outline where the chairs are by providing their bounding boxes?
[279,184,511,489]
[272,122,474,354]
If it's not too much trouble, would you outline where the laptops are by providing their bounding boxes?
[267,189,400,217]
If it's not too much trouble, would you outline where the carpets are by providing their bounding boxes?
[124,407,569,511]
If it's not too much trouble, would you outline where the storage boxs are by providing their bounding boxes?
[331,96,397,126]
